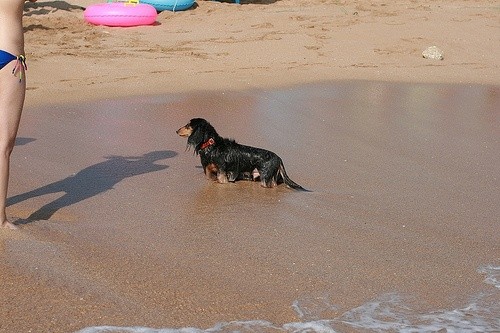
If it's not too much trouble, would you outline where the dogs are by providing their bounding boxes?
[176,118,312,192]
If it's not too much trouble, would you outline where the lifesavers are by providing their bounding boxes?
[83,2,157,26]
[139,0,196,12]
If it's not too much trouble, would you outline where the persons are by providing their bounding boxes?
[0,0,36,229]
